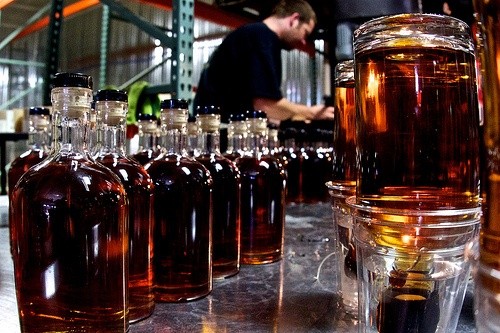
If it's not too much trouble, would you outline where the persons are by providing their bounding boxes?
[193,0,334,131]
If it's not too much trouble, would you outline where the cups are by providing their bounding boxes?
[326,12,500,333]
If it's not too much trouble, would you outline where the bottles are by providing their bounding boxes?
[8,72,334,333]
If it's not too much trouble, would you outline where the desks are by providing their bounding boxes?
[0,205,476,333]
